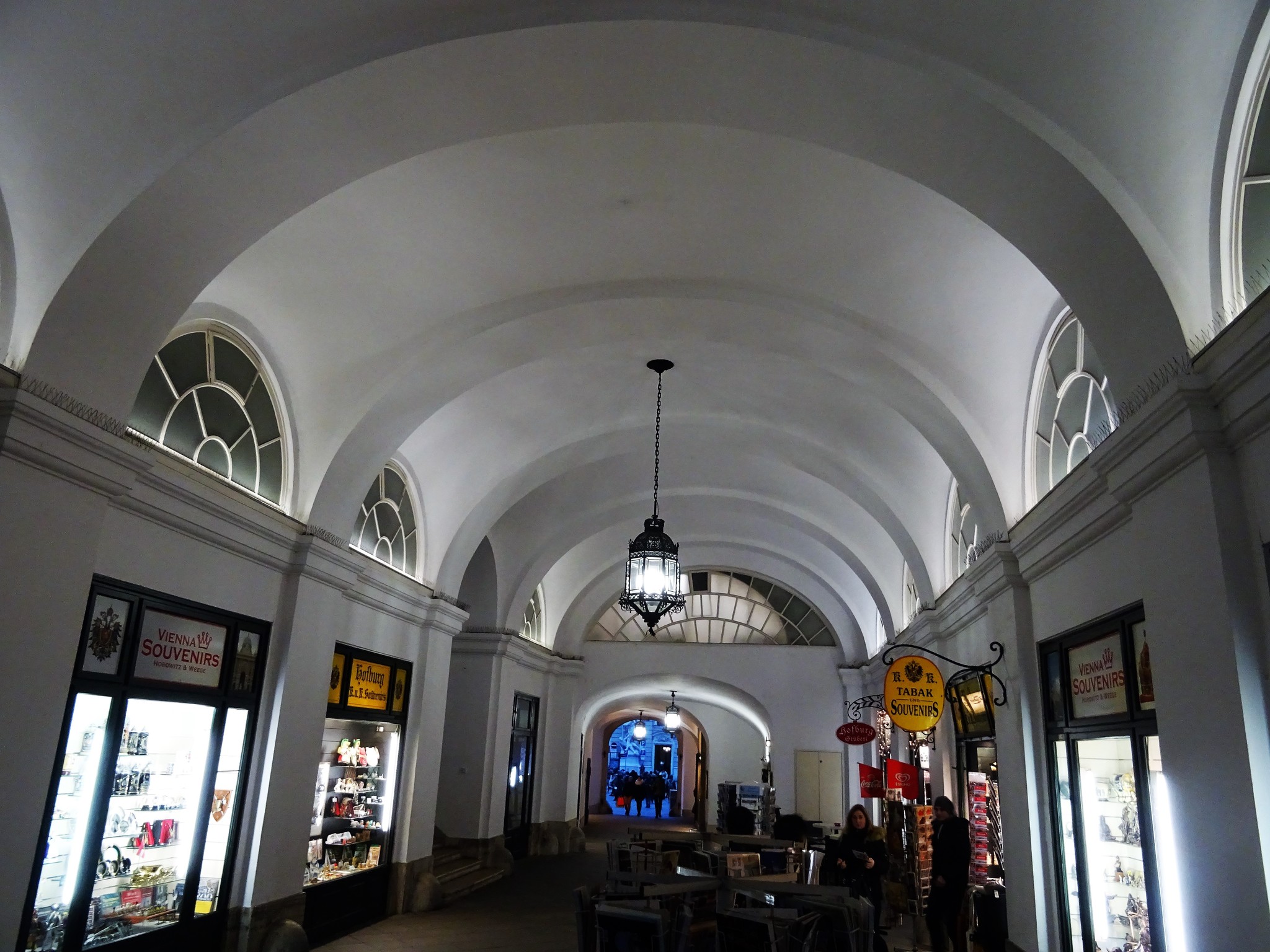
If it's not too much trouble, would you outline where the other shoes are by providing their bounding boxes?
[625,810,630,816]
[659,814,662,817]
[637,813,641,816]
[655,815,658,818]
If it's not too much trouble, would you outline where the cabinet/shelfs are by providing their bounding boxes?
[326,761,381,847]
[36,745,189,909]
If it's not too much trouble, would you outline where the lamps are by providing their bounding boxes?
[880,641,1008,724]
[617,358,687,639]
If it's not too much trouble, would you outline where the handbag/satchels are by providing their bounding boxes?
[615,796,624,808]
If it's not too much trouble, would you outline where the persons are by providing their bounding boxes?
[925,796,972,952]
[608,766,676,819]
[832,804,891,952]
